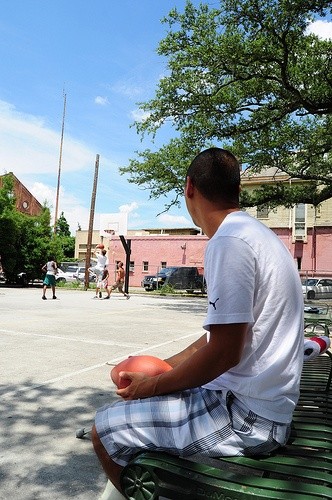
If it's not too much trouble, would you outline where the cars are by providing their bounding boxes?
[140,267,207,292]
[301,278,332,300]
[55,262,95,287]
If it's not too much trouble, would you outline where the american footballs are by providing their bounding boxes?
[110,355,174,389]
[96,244,104,249]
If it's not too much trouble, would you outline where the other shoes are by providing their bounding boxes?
[99,292,102,299]
[126,294,131,300]
[104,296,109,299]
[53,296,57,299]
[42,296,47,300]
[92,296,98,299]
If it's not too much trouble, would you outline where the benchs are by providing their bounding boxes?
[118,317,332,500]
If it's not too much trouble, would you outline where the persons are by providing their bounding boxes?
[88,245,110,299]
[91,147,304,500]
[41,256,57,300]
[104,262,130,300]
